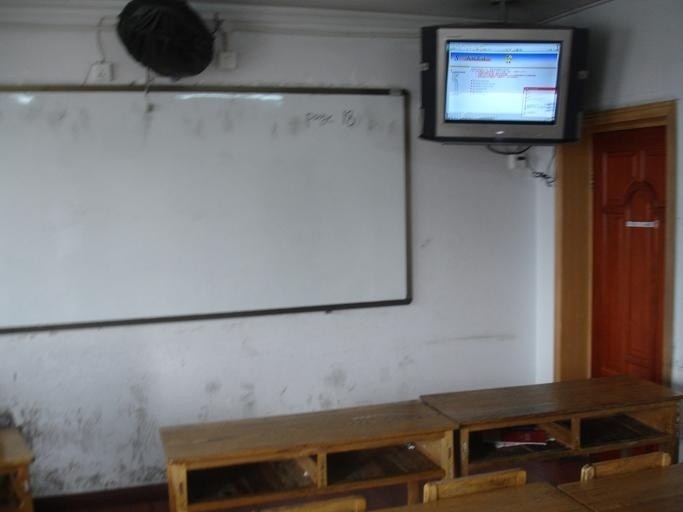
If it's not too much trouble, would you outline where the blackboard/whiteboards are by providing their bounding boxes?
[2,81,414,334]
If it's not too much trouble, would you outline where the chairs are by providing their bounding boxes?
[284,451,683,512]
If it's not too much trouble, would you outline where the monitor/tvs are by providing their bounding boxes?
[419,22,575,146]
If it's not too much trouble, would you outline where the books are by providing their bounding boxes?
[493,428,548,450]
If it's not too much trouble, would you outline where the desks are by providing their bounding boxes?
[422,378,682,477]
[161,400,461,512]
